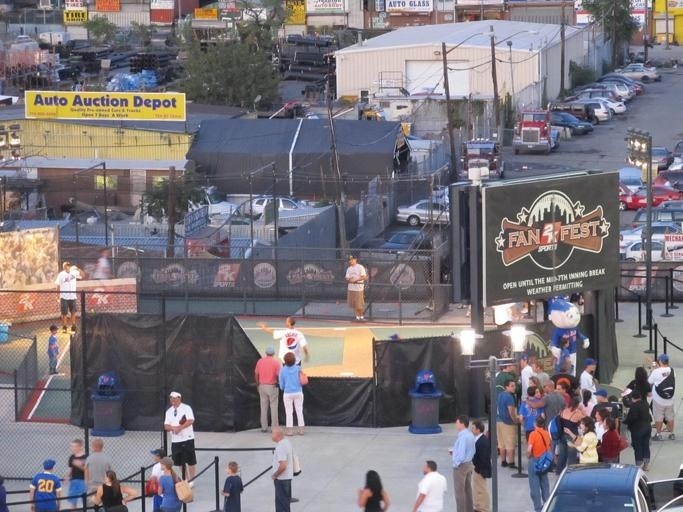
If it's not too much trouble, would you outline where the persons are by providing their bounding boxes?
[255,315,311,366]
[356,469,391,511]
[52,260,87,333]
[343,255,370,321]
[448,352,677,512]
[219,458,245,511]
[410,460,448,512]
[0,435,196,511]
[268,426,295,511]
[46,325,63,376]
[253,345,282,432]
[277,351,306,437]
[162,390,199,487]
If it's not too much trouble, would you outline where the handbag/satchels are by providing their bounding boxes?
[655,369,677,399]
[174,480,194,504]
[145,481,159,496]
[617,436,628,450]
[299,372,310,385]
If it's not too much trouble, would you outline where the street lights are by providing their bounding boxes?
[621,127,657,330]
[440,31,496,182]
[449,326,483,453]
[502,324,536,480]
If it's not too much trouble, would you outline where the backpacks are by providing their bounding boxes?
[533,450,556,475]
[546,416,560,440]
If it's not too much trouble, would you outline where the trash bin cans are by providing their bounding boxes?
[0,324,8,343]
[90,375,125,436]
[408,369,443,434]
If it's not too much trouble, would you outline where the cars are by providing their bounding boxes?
[243,195,300,218]
[0,193,240,227]
[538,459,683,512]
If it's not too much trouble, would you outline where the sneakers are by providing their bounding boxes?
[651,433,664,441]
[501,460,519,470]
[49,368,58,374]
[297,425,306,435]
[668,432,675,440]
[283,427,294,437]
[63,324,77,332]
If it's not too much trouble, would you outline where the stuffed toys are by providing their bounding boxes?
[545,297,591,377]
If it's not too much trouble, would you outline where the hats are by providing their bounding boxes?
[50,325,58,331]
[518,354,529,361]
[583,359,597,367]
[169,391,181,398]
[150,448,163,458]
[657,355,669,363]
[593,389,608,398]
[348,254,358,260]
[499,358,513,366]
[42,458,57,469]
[265,347,275,355]
